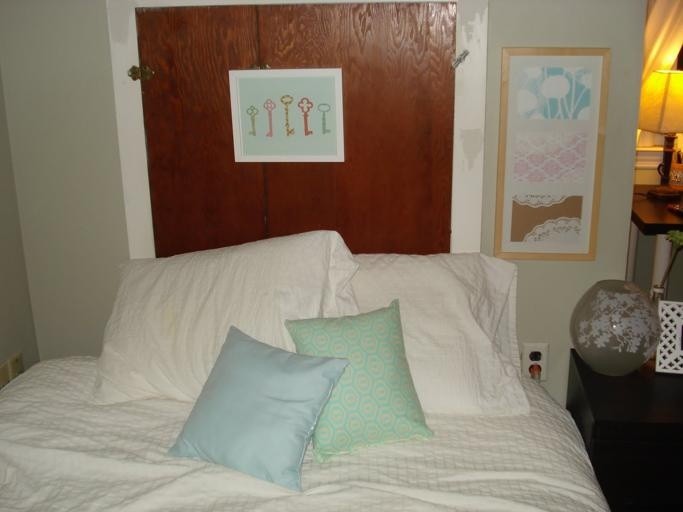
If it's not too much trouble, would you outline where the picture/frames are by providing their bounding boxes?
[227,65,346,165]
[493,46,612,261]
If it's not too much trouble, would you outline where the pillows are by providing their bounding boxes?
[345,246,527,422]
[91,230,358,404]
[282,298,435,466]
[164,323,347,496]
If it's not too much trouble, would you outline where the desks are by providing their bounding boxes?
[625,187,682,307]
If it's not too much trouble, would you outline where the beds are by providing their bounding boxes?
[1,355,609,512]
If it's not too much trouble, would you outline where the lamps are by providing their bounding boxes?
[633,67,683,202]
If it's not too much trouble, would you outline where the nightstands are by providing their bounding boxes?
[560,347,681,512]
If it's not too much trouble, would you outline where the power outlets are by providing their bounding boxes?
[522,342,551,384]
